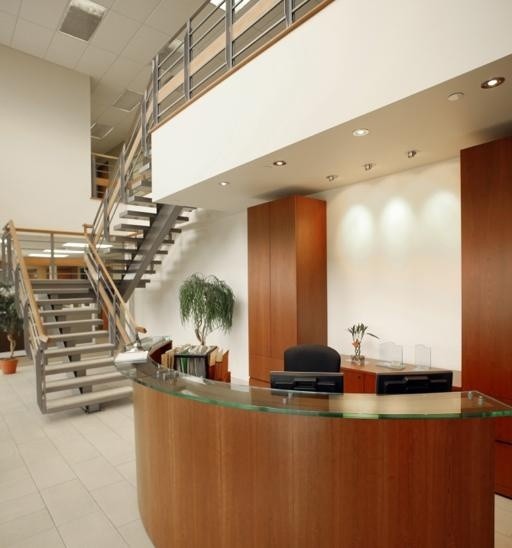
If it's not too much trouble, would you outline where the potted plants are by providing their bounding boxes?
[347,322,380,362]
[0,281,23,374]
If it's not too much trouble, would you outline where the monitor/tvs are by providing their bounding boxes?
[375,370,454,395]
[269,368,346,394]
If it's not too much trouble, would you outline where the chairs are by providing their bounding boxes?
[283,343,341,373]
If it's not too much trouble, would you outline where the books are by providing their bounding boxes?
[160,344,229,382]
[114,349,149,364]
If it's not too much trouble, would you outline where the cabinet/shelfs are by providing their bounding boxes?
[247,194,327,388]
[340,367,462,393]
[460,134,512,500]
